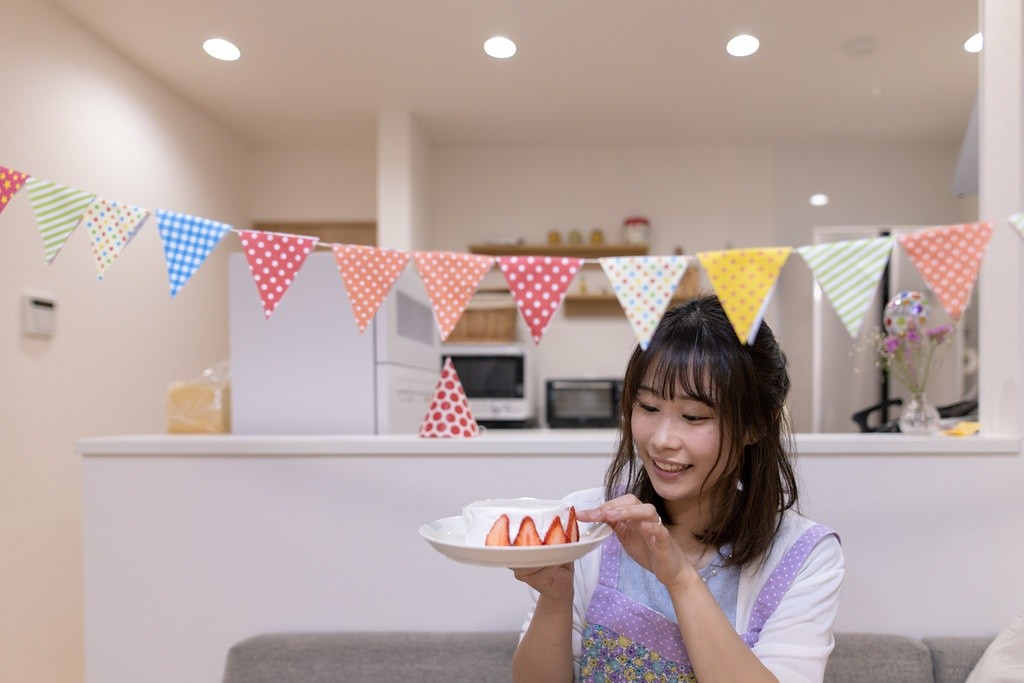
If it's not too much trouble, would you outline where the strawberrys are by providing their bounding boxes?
[484,506,579,547]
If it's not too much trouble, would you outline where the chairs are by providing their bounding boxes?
[852,396,978,434]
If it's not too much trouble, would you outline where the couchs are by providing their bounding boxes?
[224,629,1023,683]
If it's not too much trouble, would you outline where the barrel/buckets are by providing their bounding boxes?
[625,218,650,246]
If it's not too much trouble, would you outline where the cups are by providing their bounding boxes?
[548,230,562,245]
[589,229,603,244]
[569,230,581,247]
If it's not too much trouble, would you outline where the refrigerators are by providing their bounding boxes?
[229,250,440,433]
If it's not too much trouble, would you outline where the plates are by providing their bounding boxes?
[418,514,617,569]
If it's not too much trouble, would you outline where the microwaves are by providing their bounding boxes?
[542,378,623,431]
[438,347,532,422]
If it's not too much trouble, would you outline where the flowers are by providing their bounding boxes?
[848,313,957,427]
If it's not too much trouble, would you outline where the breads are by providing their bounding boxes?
[167,380,232,434]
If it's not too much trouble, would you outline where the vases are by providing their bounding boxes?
[898,389,940,435]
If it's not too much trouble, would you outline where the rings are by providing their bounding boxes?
[657,513,662,525]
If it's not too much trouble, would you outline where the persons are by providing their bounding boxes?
[511,295,844,683]
